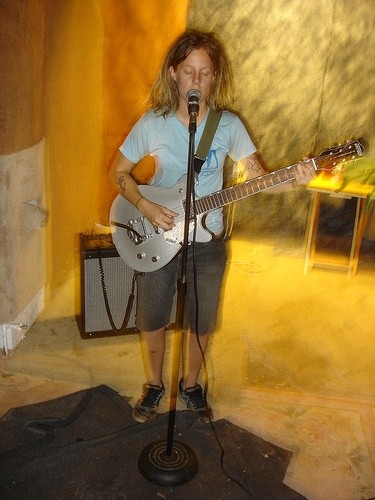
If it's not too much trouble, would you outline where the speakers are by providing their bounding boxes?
[77,233,176,338]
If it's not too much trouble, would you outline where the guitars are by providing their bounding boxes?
[109,137,369,273]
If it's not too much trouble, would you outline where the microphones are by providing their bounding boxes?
[186,89,201,133]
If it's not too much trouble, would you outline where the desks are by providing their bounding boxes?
[302,171,375,278]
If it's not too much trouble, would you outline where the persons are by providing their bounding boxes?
[109,27,317,423]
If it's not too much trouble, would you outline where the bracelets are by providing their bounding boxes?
[135,197,143,207]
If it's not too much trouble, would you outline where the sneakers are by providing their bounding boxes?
[133,379,166,420]
[177,376,213,418]
